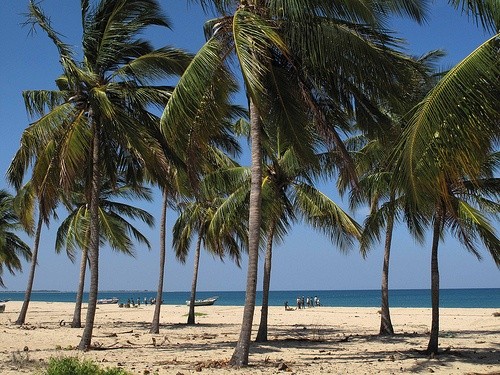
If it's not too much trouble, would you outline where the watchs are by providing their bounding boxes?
[297,295,320,309]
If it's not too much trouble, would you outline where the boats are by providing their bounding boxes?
[97,297,119,304]
[185,298,216,306]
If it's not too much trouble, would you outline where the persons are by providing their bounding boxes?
[284,301,288,310]
[119,296,155,308]
